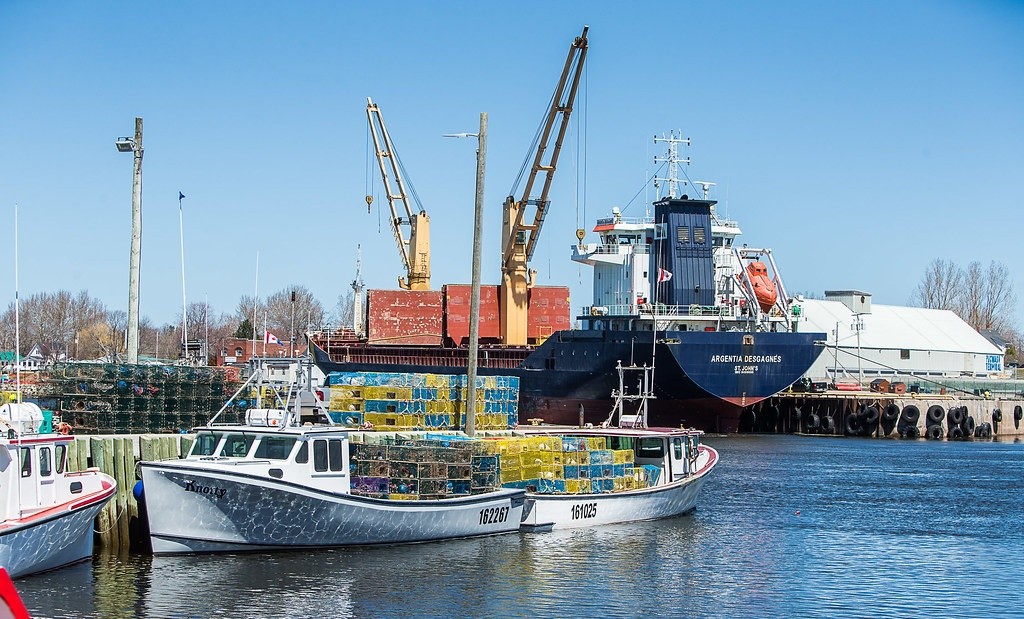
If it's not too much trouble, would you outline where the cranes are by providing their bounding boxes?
[499,22,590,348]
[363,96,432,291]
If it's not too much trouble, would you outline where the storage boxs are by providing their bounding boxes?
[366,289,443,346]
[442,284,570,347]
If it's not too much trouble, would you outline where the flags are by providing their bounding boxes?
[657,268,673,282]
[264,331,284,346]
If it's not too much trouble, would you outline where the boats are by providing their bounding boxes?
[132,289,528,558]
[502,303,720,535]
[298,124,833,439]
[0,200,118,583]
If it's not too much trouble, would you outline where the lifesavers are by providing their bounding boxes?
[948,408,963,425]
[767,404,783,424]
[947,426,963,438]
[994,409,1002,422]
[858,403,879,424]
[901,425,920,440]
[750,410,757,426]
[975,423,992,437]
[927,424,943,439]
[1014,405,1023,420]
[883,404,898,420]
[62,426,68,435]
[821,415,834,432]
[928,405,945,422]
[902,405,919,423]
[792,407,802,419]
[961,405,974,435]
[807,414,820,429]
[845,412,863,434]
